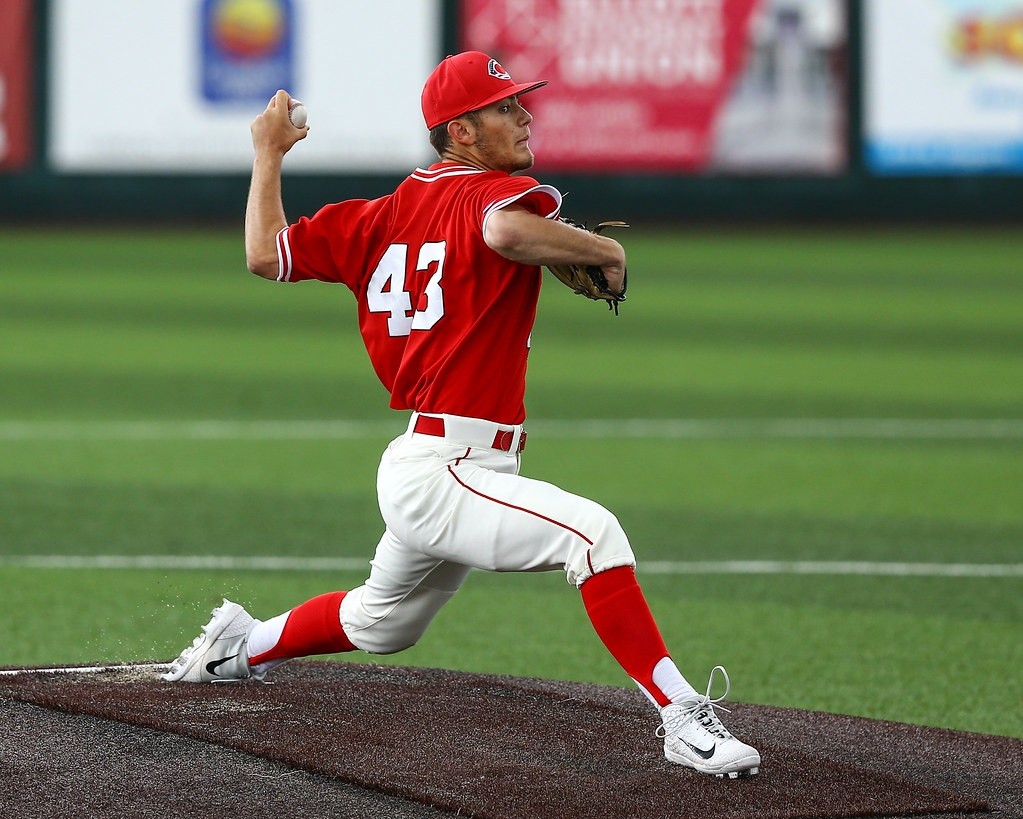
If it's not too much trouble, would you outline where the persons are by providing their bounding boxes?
[164,51,762,775]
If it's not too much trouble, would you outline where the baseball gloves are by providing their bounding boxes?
[546,216,628,301]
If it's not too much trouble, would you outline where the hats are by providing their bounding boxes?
[420,50,548,130]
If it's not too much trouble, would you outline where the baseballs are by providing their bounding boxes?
[288,98,307,129]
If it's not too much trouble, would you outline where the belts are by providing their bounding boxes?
[413,415,528,454]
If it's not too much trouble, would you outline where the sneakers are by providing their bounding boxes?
[161,597,268,684]
[659,695,761,780]
